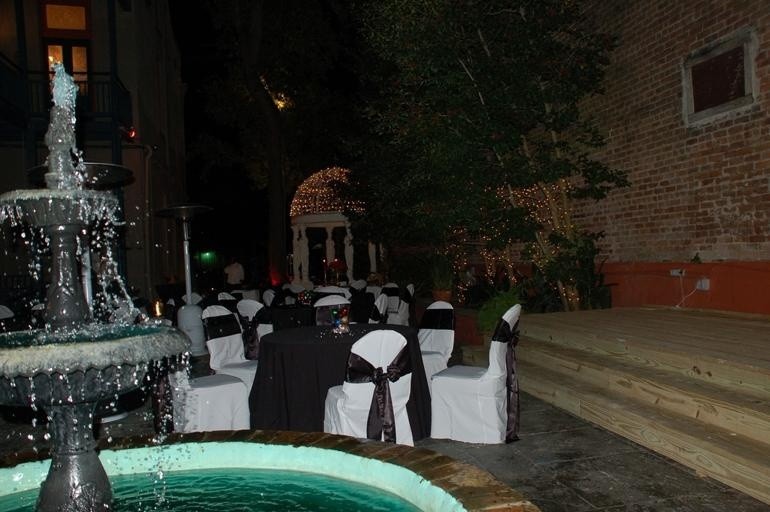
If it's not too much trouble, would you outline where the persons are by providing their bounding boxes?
[224,257,246,292]
